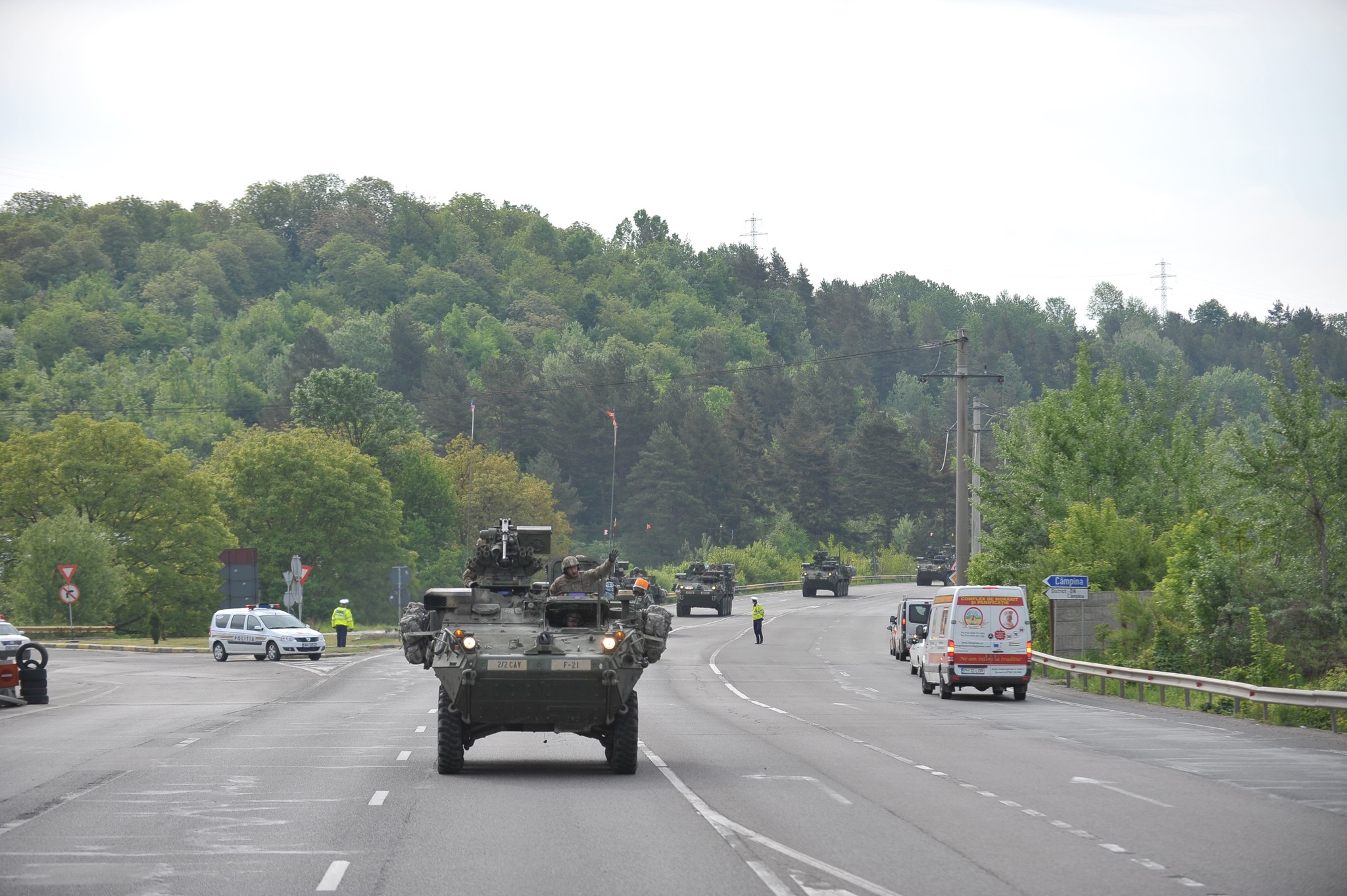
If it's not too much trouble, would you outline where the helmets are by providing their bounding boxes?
[633,567,640,574]
[561,556,584,575]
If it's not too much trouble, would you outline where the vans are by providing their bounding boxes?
[889,594,934,661]
[915,583,1034,702]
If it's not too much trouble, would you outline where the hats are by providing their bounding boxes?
[339,599,349,604]
[751,596,759,601]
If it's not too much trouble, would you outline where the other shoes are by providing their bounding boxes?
[755,642,760,644]
[759,639,764,644]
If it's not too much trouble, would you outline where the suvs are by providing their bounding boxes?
[207,603,326,662]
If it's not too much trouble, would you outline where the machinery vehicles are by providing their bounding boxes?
[670,560,739,617]
[800,551,857,598]
[621,563,668,606]
[395,515,674,775]
[914,544,956,586]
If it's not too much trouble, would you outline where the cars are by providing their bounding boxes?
[886,620,897,656]
[906,625,927,678]
[0,614,35,662]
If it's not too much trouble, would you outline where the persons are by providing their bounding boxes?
[1002,611,1014,629]
[751,597,764,644]
[633,567,643,577]
[331,599,353,647]
[709,563,717,571]
[549,549,620,627]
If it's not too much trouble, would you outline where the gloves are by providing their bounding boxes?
[609,549,620,562]
[348,628,353,632]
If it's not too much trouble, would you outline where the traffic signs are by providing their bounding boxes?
[1042,587,1089,600]
[1042,575,1089,588]
